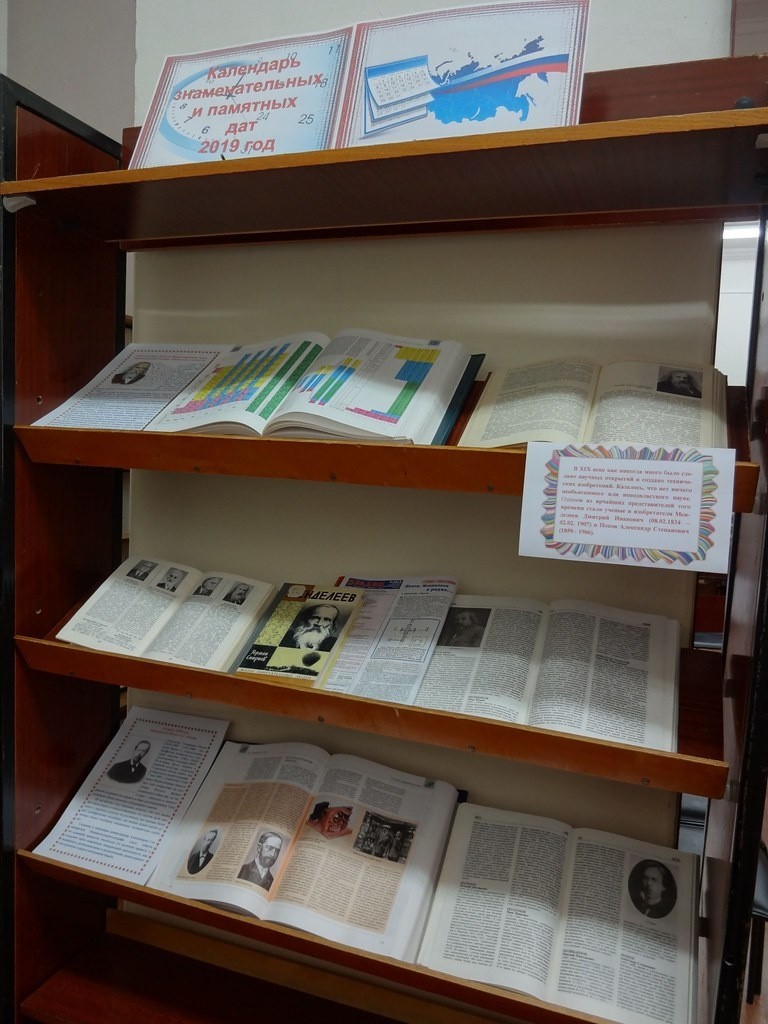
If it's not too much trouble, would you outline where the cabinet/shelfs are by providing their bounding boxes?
[0,52,768,1024]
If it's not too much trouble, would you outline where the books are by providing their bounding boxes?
[29,326,729,1024]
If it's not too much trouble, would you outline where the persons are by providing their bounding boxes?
[222,583,249,605]
[107,740,151,783]
[187,830,217,874]
[156,569,183,593]
[192,577,220,596]
[111,362,150,385]
[657,370,702,398]
[237,832,282,892]
[126,561,153,581]
[437,608,485,648]
[279,604,339,652]
[630,861,672,919]
